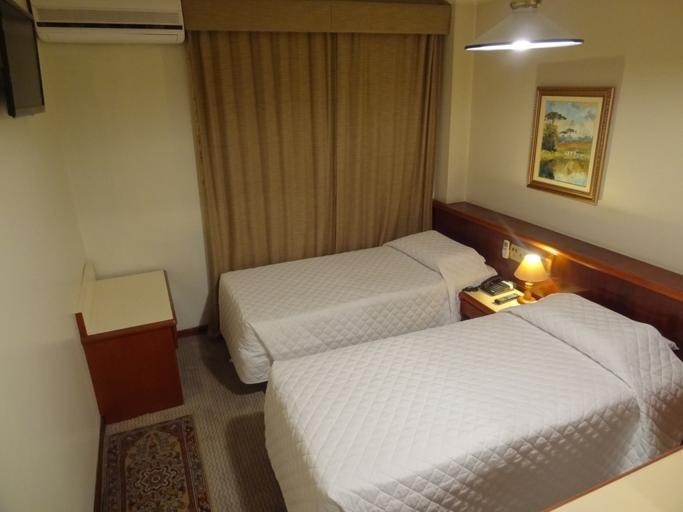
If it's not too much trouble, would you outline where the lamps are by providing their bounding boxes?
[462,1,585,54]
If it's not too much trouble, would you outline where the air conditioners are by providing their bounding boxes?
[31,0,188,46]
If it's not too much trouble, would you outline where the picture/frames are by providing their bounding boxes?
[525,84,616,205]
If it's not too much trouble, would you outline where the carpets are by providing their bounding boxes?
[99,415,212,512]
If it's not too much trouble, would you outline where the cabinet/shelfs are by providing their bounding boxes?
[76,257,184,423]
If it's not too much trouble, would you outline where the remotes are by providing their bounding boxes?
[495,292,519,304]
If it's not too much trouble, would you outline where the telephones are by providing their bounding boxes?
[481,274,510,296]
